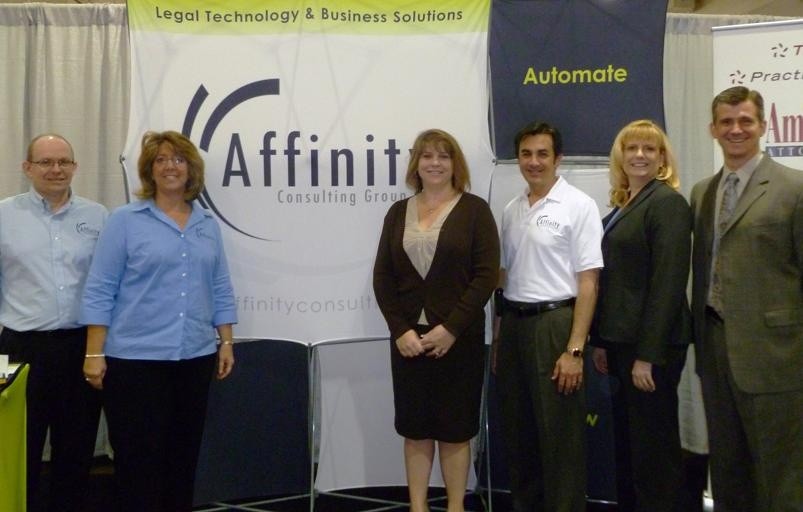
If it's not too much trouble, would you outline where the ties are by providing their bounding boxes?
[712,174,738,320]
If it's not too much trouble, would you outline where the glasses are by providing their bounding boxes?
[28,158,74,168]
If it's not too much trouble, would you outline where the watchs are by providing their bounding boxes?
[565,345,582,358]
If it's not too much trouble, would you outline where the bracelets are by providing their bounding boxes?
[82,352,106,357]
[218,338,233,345]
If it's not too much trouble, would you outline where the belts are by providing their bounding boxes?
[502,298,574,316]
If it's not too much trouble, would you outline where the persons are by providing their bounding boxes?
[687,86,802,511]
[372,127,499,512]
[493,119,605,511]
[589,119,691,509]
[1,132,110,511]
[75,130,236,509]
